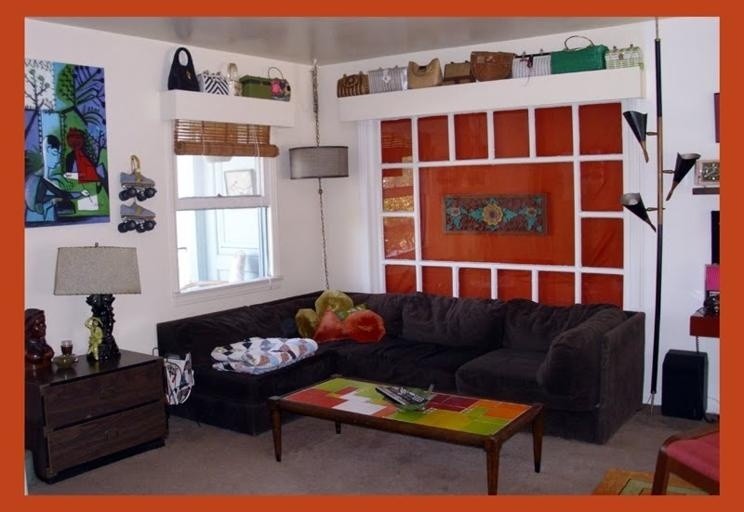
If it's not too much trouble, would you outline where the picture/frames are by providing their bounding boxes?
[693,158,720,188]
[441,191,547,237]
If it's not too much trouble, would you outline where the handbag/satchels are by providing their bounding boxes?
[198,68,229,95]
[335,58,445,98]
[268,66,293,102]
[446,35,645,87]
[227,63,246,96]
[167,47,200,92]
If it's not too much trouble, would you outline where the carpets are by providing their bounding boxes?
[590,467,710,496]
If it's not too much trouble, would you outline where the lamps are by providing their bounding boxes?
[622,36,700,406]
[284,52,352,179]
[54,240,144,362]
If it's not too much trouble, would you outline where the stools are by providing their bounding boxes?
[653,431,719,495]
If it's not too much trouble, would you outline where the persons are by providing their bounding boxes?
[85,316,103,361]
[25,309,54,372]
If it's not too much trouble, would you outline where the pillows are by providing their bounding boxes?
[294,289,387,344]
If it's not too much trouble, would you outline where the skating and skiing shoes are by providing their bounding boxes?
[118,202,157,232]
[119,171,158,202]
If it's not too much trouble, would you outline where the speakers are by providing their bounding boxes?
[660,349,708,420]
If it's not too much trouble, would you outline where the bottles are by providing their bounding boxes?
[52,340,78,370]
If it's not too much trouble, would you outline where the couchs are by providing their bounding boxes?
[156,289,648,445]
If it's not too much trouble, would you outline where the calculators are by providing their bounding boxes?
[376,386,427,406]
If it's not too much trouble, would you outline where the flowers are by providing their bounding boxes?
[446,198,542,231]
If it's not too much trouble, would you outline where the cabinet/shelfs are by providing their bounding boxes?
[25,348,169,485]
